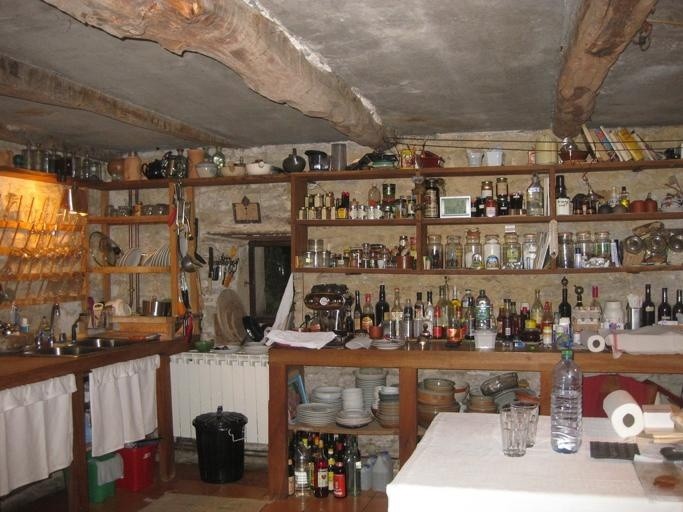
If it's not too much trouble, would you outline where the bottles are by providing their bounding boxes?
[525,174,544,216]
[0,142,142,183]
[550,348,584,452]
[286,431,390,500]
[354,278,682,347]
[423,179,439,217]
[173,149,187,178]
[367,183,381,205]
[555,175,569,215]
[38,316,52,338]
[19,317,30,334]
[298,228,610,270]
[608,185,630,209]
[104,203,169,217]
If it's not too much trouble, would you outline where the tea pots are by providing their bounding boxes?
[305,150,331,171]
[141,159,162,178]
[282,147,304,173]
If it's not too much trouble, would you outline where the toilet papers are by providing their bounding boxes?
[603,390,644,437]
[587,335,605,353]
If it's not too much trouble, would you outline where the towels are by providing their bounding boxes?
[94,455,123,487]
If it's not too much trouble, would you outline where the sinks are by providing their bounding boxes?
[24,347,105,358]
[78,338,135,349]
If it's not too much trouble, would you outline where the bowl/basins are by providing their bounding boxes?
[467,153,483,167]
[417,373,520,430]
[484,151,503,167]
[196,165,217,177]
[194,340,213,353]
[558,151,590,163]
[473,330,498,349]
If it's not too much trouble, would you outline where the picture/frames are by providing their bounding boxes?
[438,195,472,220]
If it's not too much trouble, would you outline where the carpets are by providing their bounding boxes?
[130,488,275,511]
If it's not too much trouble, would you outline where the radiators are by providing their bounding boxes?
[168,349,268,447]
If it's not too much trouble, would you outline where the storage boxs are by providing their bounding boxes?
[61,450,115,502]
[112,444,155,492]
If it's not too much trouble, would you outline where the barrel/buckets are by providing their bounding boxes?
[372,451,392,492]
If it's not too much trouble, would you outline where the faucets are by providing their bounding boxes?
[50,304,61,339]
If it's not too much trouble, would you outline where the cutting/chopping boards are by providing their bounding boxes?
[216,289,248,341]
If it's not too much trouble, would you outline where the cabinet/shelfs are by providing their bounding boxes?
[266,343,407,500]
[553,157,683,274]
[556,347,682,418]
[288,165,421,273]
[87,181,195,334]
[0,218,87,307]
[408,342,556,467]
[422,164,554,275]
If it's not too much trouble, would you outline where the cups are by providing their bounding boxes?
[509,400,538,448]
[330,144,347,170]
[498,407,529,457]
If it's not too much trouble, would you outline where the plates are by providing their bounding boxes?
[105,299,131,316]
[212,344,268,355]
[117,242,172,267]
[370,340,404,350]
[297,368,398,429]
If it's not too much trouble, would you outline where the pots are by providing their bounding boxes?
[219,162,246,176]
[245,162,271,174]
[143,298,171,316]
[238,316,263,346]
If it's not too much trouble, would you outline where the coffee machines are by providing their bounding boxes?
[304,285,352,344]
